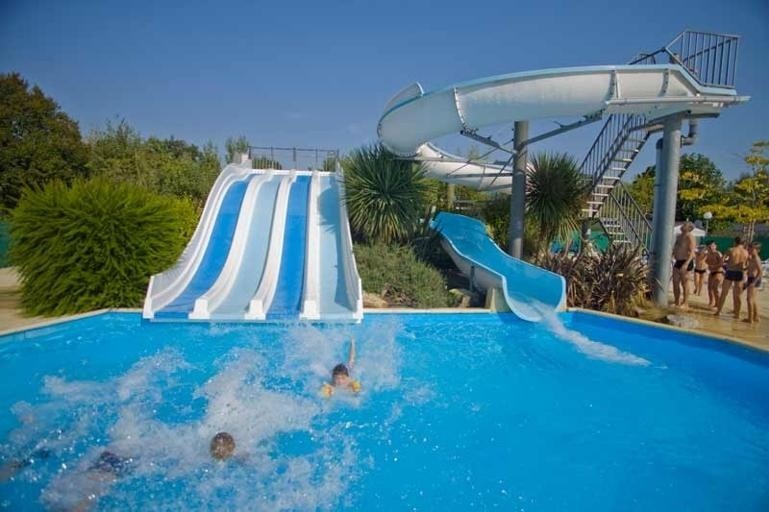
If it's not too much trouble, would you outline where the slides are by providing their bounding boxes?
[377,64,737,322]
[142,159,363,324]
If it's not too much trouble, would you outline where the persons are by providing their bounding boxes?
[210,432,236,458]
[312,337,361,397]
[44,449,131,512]
[694,235,762,324]
[671,221,695,310]
[0,442,55,478]
